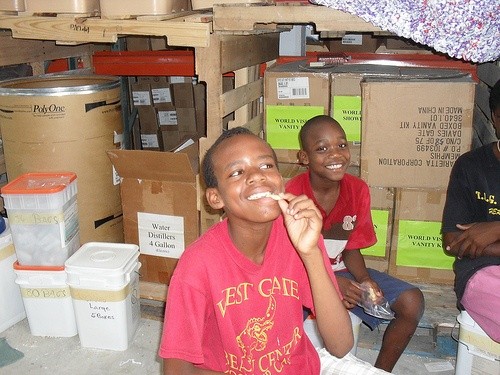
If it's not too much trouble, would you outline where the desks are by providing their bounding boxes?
[0,12,280,237]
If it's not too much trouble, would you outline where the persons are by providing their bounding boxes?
[440,79,500,344]
[282,115,425,373]
[159,127,394,375]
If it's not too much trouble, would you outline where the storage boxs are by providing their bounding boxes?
[0,0,500,375]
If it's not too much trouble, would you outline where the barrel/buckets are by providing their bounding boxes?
[13,260,78,338]
[302,309,362,356]
[0,73,125,247]
[64,242,144,350]
[1,173,80,267]
[451,309,500,374]
